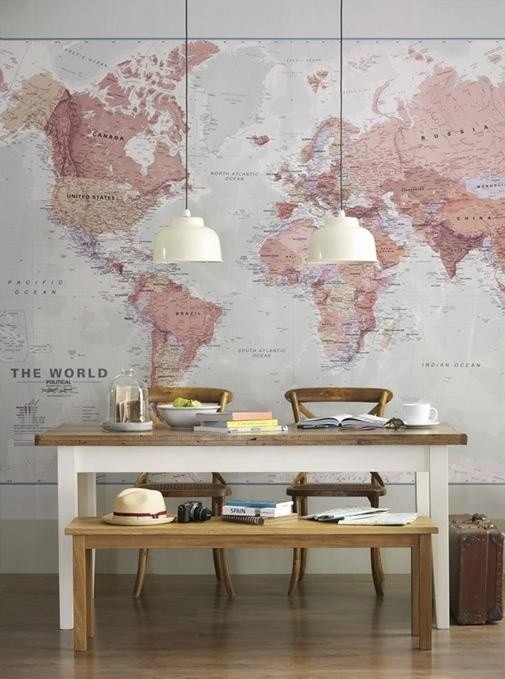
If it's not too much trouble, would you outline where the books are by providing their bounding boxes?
[194,412,392,434]
[222,498,298,525]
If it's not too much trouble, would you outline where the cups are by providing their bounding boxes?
[403,402,440,423]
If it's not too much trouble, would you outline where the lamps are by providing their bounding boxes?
[307,0,377,267]
[152,0,225,268]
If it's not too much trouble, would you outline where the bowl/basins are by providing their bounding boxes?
[157,404,222,430]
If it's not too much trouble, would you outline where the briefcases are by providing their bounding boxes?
[450,512,505,626]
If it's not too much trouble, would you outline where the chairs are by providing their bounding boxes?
[132,385,239,599]
[284,385,393,600]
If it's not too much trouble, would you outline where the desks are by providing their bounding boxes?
[30,417,470,631]
[60,514,443,651]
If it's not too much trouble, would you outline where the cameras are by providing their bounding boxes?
[177,499,212,524]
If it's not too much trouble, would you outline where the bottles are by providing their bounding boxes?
[108,369,147,423]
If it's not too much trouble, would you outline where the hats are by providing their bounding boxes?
[101,487,176,527]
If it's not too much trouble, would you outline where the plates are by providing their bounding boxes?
[102,422,153,431]
[399,421,440,429]
